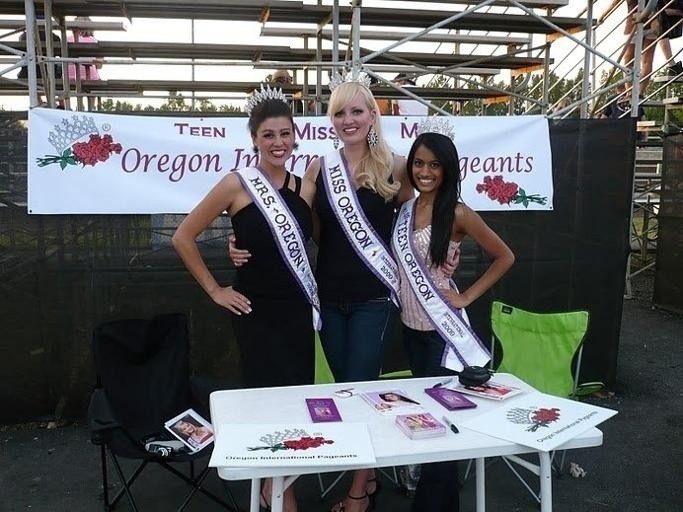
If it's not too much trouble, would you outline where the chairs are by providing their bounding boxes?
[313,331,412,499]
[87,313,242,512]
[463,300,590,504]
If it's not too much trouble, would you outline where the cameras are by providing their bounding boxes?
[459,366,491,385]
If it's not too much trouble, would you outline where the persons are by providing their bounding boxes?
[368,73,392,116]
[270,69,315,114]
[552,0,682,149]
[174,420,213,448]
[66,16,104,111]
[229,68,419,511]
[17,14,65,109]
[172,86,319,512]
[390,133,516,512]
[392,74,429,115]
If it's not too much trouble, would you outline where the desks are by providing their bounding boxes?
[211,373,602,512]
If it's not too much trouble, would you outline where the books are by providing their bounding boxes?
[366,379,522,441]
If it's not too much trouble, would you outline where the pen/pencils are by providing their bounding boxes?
[433,378,452,388]
[443,416,459,434]
